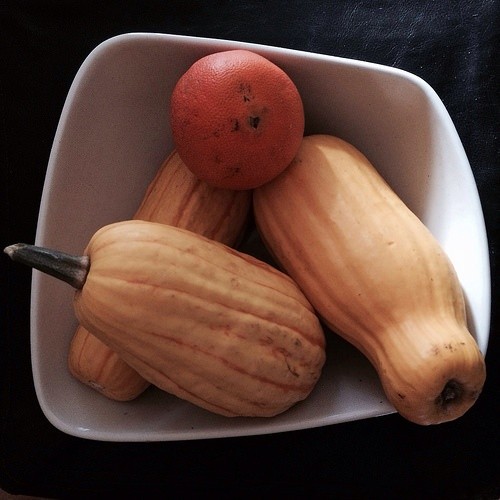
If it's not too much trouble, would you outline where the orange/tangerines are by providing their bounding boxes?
[172,49,305,192]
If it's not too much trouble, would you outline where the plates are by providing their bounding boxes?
[28,31,492,442]
